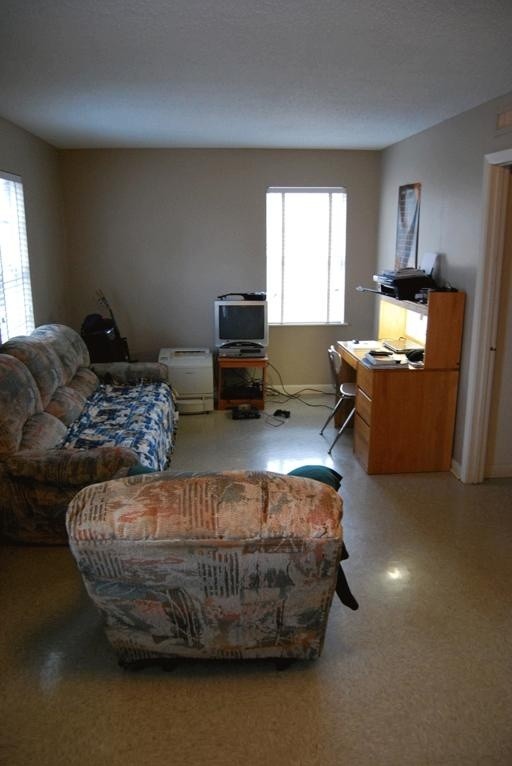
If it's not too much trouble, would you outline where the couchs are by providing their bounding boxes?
[0,323,178,545]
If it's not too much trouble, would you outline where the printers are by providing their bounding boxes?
[157,346,215,413]
[356,268,433,300]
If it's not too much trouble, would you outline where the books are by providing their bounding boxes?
[363,350,410,371]
[372,267,427,285]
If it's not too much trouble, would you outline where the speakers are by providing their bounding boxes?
[80,341,123,363]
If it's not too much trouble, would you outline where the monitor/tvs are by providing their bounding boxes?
[212,298,269,348]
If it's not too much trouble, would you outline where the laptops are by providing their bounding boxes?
[382,339,425,353]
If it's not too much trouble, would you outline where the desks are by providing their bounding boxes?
[338,340,459,475]
[215,353,269,410]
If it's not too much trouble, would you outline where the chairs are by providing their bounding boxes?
[64,468,343,671]
[319,345,356,454]
